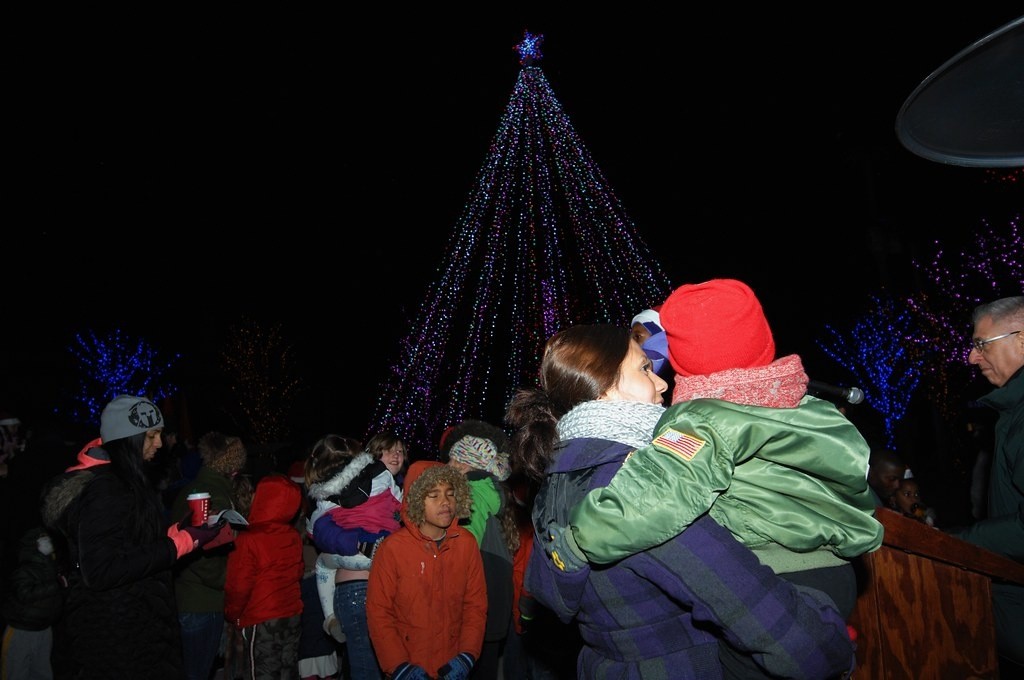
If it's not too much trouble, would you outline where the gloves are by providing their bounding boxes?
[436,652,476,680]
[391,662,434,680]
[518,614,535,632]
[165,509,227,560]
[541,521,587,573]
[356,534,386,560]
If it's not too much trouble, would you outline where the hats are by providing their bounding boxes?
[630,304,665,335]
[99,394,165,444]
[0,414,20,425]
[659,278,776,376]
[288,461,307,483]
[448,435,512,482]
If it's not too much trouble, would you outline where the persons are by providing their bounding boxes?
[0,296,938,679]
[942,295,1023,680]
[501,324,857,680]
[546,276,886,680]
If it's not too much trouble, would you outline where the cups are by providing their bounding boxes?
[186,493,211,527]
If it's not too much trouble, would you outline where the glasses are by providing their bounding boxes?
[969,329,1020,351]
[383,449,403,456]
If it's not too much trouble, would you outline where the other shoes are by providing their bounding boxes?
[323,613,346,643]
